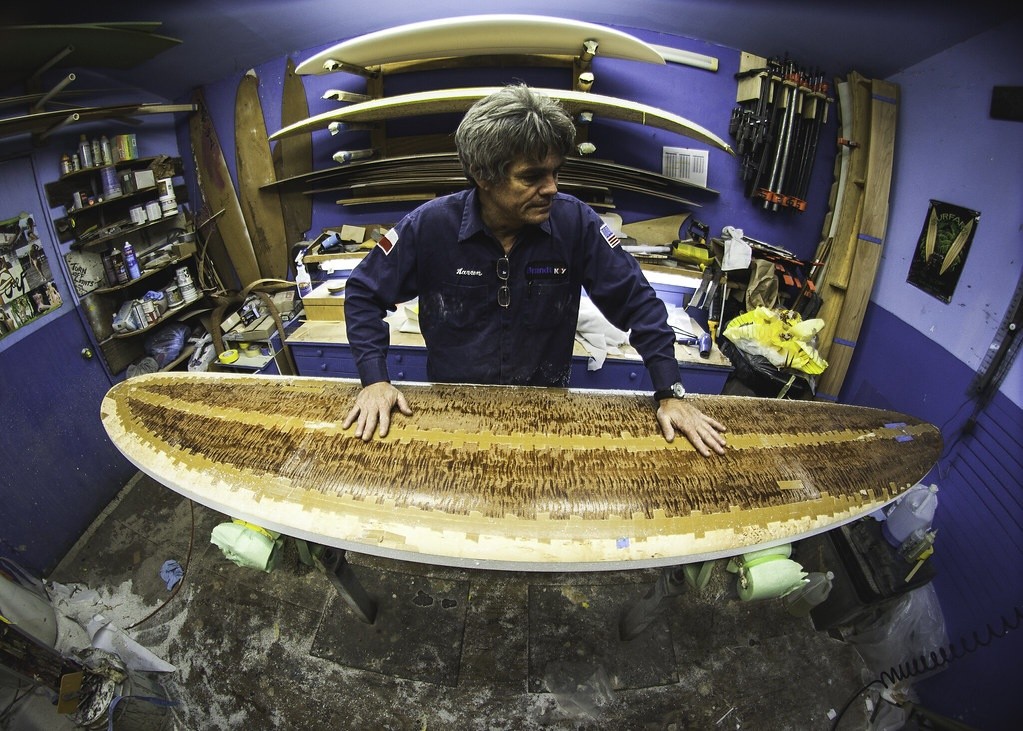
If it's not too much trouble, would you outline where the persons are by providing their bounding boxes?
[341,85,728,456]
[0,218,60,335]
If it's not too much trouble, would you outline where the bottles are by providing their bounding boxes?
[131,300,148,330]
[60,134,112,175]
[101,241,144,288]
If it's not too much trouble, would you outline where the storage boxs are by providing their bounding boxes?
[787,499,939,630]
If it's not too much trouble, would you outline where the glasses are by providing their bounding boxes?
[497,255,512,309]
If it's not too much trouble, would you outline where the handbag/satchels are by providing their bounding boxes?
[723,308,828,376]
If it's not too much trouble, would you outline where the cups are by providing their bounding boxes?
[166,267,198,307]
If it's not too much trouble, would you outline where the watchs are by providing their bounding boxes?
[654,382,685,401]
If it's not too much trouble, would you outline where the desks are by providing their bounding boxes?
[719,319,808,391]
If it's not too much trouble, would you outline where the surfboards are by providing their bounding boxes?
[293,13,666,75]
[271,56,314,249]
[188,92,262,287]
[258,150,723,207]
[234,66,290,282]
[98,370,947,573]
[262,84,741,158]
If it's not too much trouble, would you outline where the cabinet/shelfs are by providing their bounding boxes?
[44,155,205,375]
[284,299,736,395]
[301,228,696,306]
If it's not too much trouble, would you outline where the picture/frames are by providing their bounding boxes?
[905,198,981,304]
